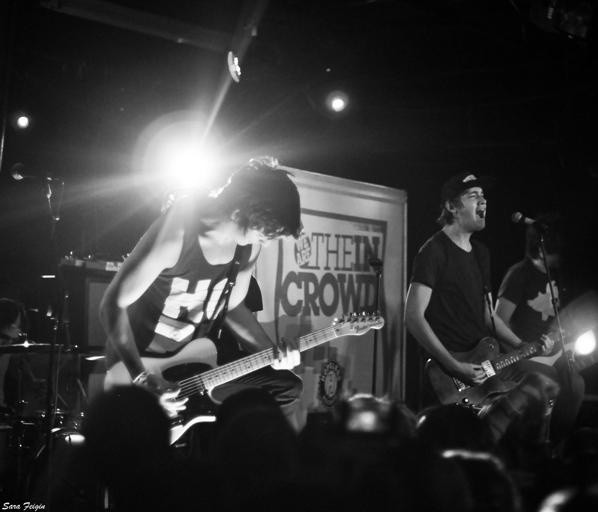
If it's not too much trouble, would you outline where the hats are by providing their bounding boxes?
[440,171,493,202]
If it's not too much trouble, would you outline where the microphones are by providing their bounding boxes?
[510,211,549,229]
[8,161,65,182]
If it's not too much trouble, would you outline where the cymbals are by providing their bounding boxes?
[0,342,80,353]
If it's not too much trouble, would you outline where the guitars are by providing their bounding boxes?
[528,341,598,395]
[424,327,565,406]
[104,311,385,446]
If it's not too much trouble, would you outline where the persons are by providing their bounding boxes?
[0,297,28,419]
[43,380,526,512]
[97,164,305,422]
[494,211,588,449]
[401,168,555,386]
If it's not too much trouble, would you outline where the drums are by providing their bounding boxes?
[24,426,109,512]
[32,408,71,456]
[7,420,35,455]
